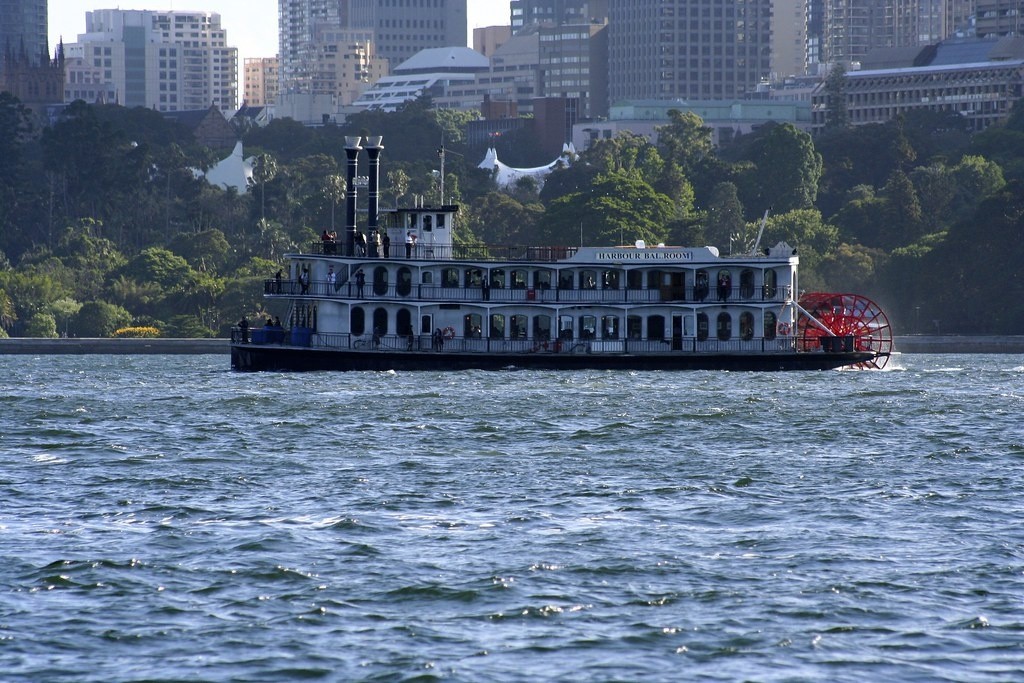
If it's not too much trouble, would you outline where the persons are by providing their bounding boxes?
[300,268,310,295]
[374,230,382,257]
[469,324,480,338]
[327,266,336,296]
[355,268,365,299]
[406,324,414,351]
[322,229,337,255]
[561,275,573,290]
[264,319,273,344]
[354,230,367,257]
[433,327,444,352]
[695,273,707,303]
[718,274,730,303]
[274,316,284,345]
[238,316,249,344]
[481,275,490,301]
[382,232,390,258]
[584,276,594,289]
[405,231,414,259]
[276,269,284,294]
[372,324,382,350]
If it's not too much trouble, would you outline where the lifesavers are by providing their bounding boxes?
[776,323,790,336]
[442,326,456,338]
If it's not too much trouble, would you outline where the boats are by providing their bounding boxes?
[229,135,903,372]
